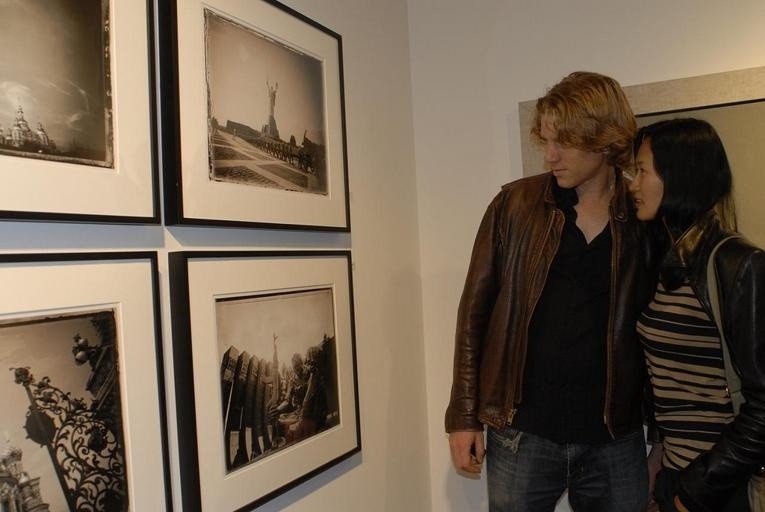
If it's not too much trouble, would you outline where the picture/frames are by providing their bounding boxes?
[158,0,351,232]
[167,250,362,511]
[0,1,160,226]
[0,250,173,511]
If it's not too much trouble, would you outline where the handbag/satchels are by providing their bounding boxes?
[745,463,763,511]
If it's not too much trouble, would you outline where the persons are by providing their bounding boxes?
[264,352,310,440]
[231,127,323,175]
[445,70,648,511]
[627,115,765,512]
[265,78,281,138]
[274,345,329,446]
